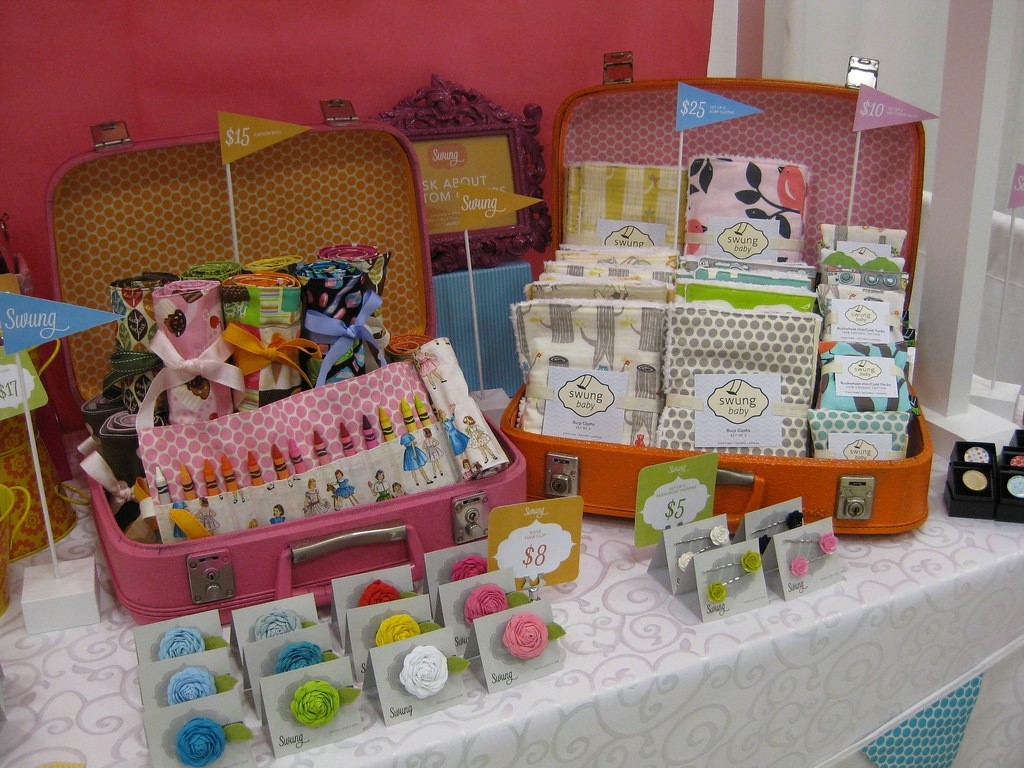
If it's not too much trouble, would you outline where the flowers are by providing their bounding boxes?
[398,644,470,699]
[758,533,772,555]
[375,614,443,646]
[289,678,361,728]
[818,532,839,554]
[275,641,340,674]
[166,665,238,707]
[254,609,318,643]
[174,716,256,768]
[741,550,762,574]
[463,583,532,627]
[158,628,230,662]
[678,551,694,572]
[502,611,567,660]
[710,526,731,546]
[358,579,419,607]
[786,509,804,530]
[790,556,809,576]
[706,581,728,604]
[451,553,488,582]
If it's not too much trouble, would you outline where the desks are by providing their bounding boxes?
[1,450,1024,768]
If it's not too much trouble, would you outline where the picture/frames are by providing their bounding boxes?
[366,74,554,272]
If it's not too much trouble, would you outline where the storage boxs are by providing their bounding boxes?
[44,99,527,627]
[499,76,935,536]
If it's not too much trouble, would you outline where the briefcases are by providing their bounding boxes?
[46,100,527,626]
[499,51,933,535]
